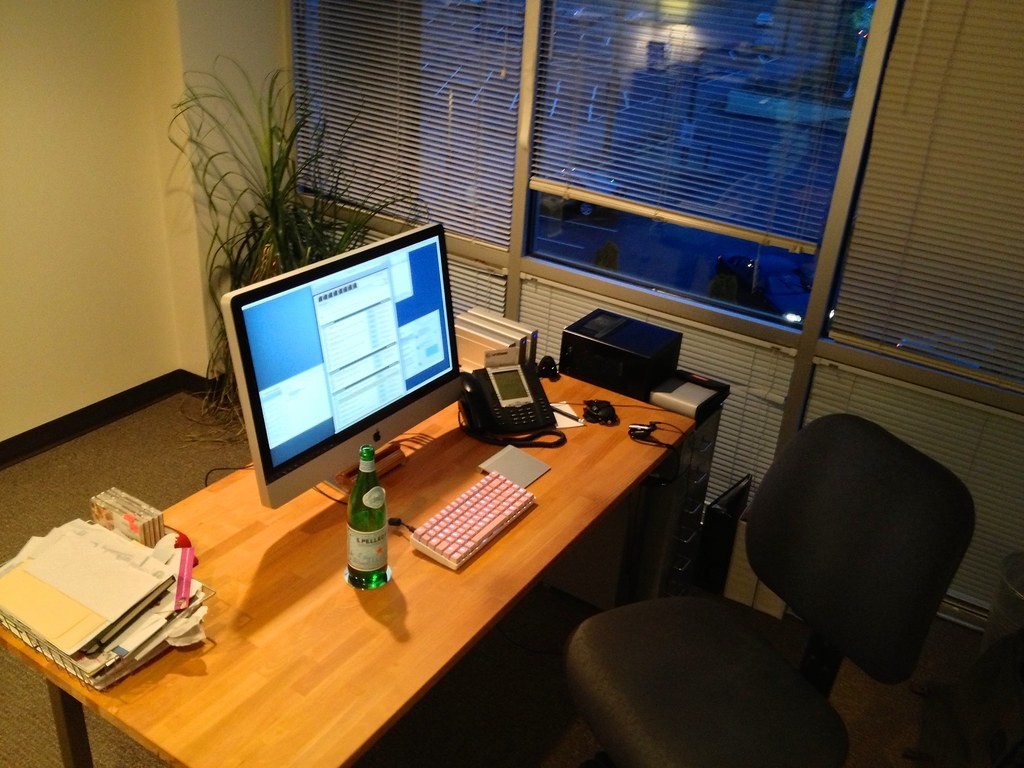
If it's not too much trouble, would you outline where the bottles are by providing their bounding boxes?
[346,445,388,590]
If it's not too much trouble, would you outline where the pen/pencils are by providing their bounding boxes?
[549,400,584,425]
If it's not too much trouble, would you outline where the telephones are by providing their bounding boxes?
[455,362,557,440]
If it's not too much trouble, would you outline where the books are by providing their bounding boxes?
[0,487,208,691]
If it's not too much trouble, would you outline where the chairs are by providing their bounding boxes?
[559,412,976,768]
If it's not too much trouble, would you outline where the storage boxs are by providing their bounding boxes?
[559,308,683,398]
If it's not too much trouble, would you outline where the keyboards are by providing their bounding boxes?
[411,470,535,571]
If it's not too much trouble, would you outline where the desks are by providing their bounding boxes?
[0,362,695,768]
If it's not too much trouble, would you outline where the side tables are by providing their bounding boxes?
[537,368,731,611]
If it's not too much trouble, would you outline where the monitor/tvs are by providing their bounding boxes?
[220,222,462,509]
